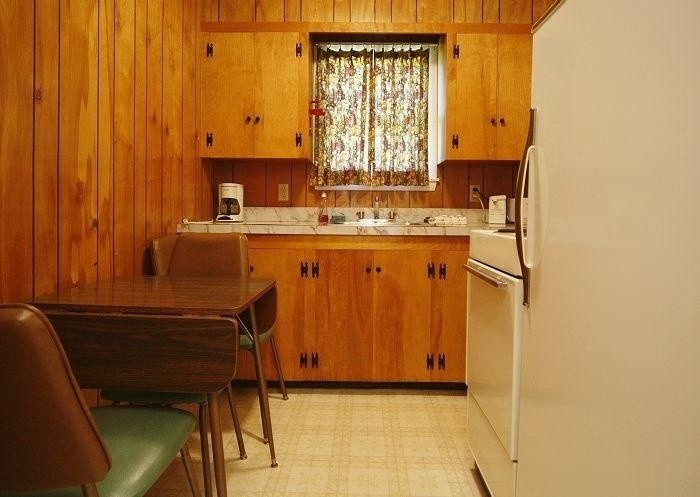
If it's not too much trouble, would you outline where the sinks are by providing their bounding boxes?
[356,217,392,224]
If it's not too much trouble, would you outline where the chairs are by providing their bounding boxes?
[1,301,196,497]
[152,233,288,411]
[164,232,270,445]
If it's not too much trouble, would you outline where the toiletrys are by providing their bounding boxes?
[318,191,328,226]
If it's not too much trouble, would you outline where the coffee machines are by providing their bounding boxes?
[216,183,244,222]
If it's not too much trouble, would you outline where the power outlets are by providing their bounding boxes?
[469,184,480,202]
[278,183,289,201]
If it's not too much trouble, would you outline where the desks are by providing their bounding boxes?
[28,274,276,497]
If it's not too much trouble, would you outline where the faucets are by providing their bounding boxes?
[372,195,381,218]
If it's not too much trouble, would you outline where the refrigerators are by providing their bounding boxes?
[514,0,700,494]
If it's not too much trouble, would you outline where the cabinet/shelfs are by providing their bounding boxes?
[310,235,435,383]
[200,22,312,166]
[438,32,533,168]
[437,236,471,382]
[237,236,309,381]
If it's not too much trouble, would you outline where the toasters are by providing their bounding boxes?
[507,197,527,223]
